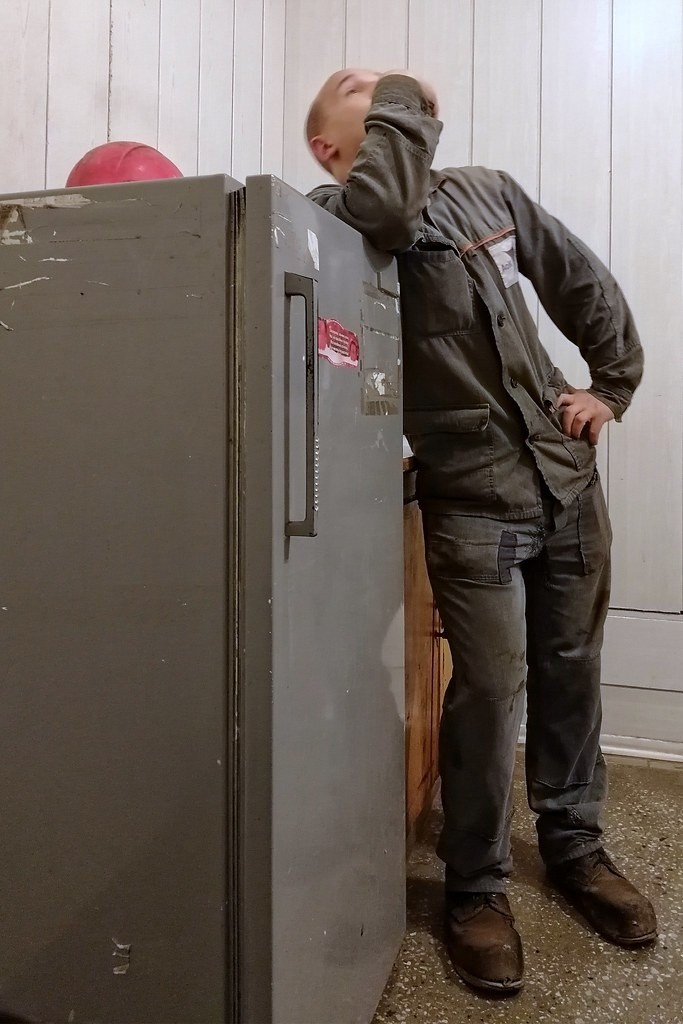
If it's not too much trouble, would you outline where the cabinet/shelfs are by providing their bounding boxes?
[403,456,453,834]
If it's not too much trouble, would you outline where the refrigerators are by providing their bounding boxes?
[1,176,407,1024]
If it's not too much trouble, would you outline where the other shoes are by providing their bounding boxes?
[445,888,526,991]
[546,847,658,943]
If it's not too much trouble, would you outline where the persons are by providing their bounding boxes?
[303,67,660,992]
